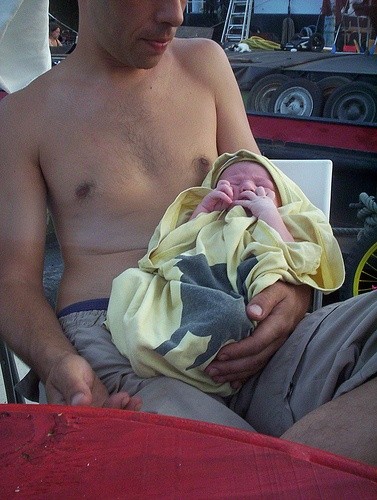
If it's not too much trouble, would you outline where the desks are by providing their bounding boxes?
[0,403,377,500]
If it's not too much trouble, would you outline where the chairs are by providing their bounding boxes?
[268,158,332,311]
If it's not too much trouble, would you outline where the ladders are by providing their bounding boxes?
[220,0,253,49]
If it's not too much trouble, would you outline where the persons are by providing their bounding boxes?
[105,149,348,398]
[1,0,376,468]
